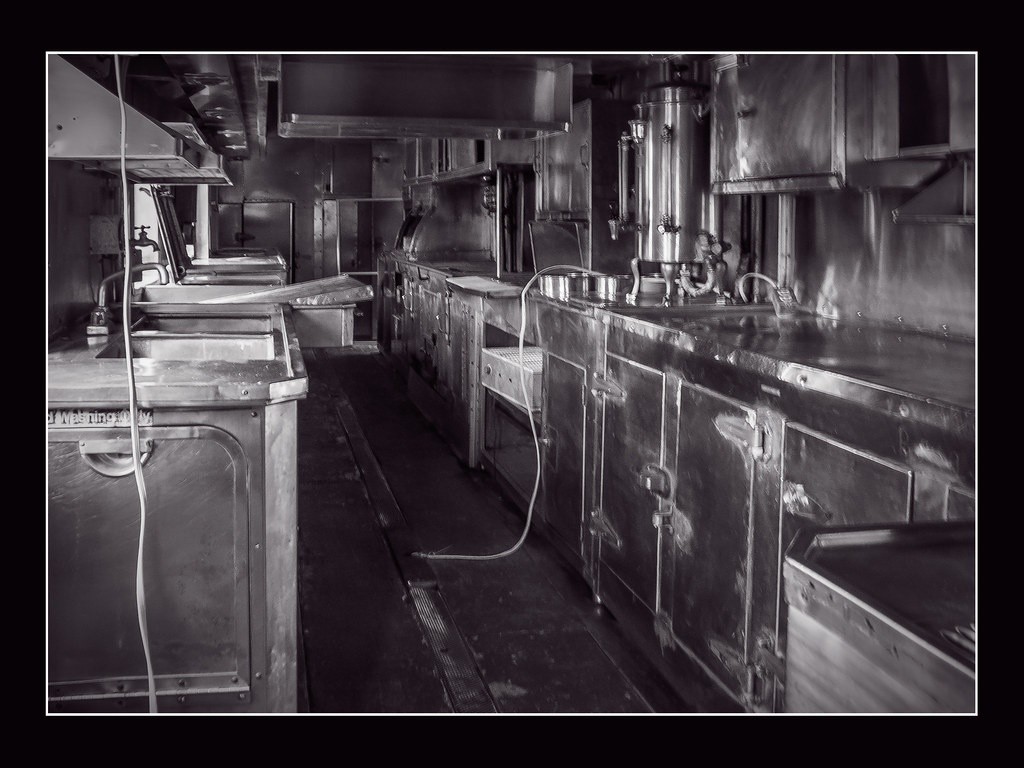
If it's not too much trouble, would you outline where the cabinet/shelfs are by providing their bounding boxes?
[546,326,974,714]
[401,273,482,468]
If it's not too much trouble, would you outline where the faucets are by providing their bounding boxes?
[129,225,161,253]
[89,263,170,326]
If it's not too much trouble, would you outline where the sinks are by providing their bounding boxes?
[132,285,281,305]
[93,333,277,362]
[128,310,278,335]
